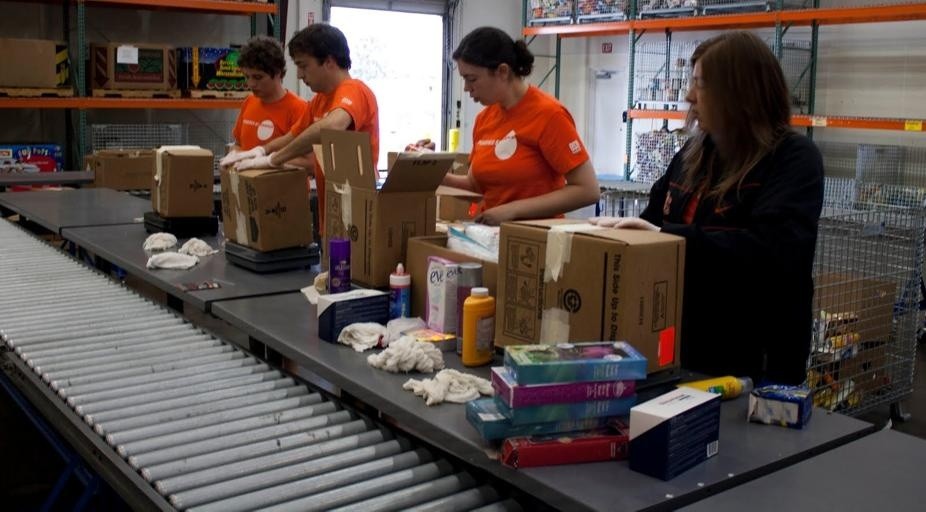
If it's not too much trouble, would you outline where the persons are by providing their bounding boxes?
[216,21,382,257]
[581,32,824,387]
[413,24,603,226]
[228,32,316,203]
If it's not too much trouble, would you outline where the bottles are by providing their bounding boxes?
[328,238,352,292]
[390,263,411,319]
[677,375,754,402]
[460,286,494,366]
[456,265,485,357]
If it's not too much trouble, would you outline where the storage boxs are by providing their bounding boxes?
[493,218,686,375]
[153,144,311,252]
[83,149,152,190]
[311,128,497,324]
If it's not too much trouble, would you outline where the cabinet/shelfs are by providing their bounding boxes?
[519,2,924,132]
[1,0,280,109]
